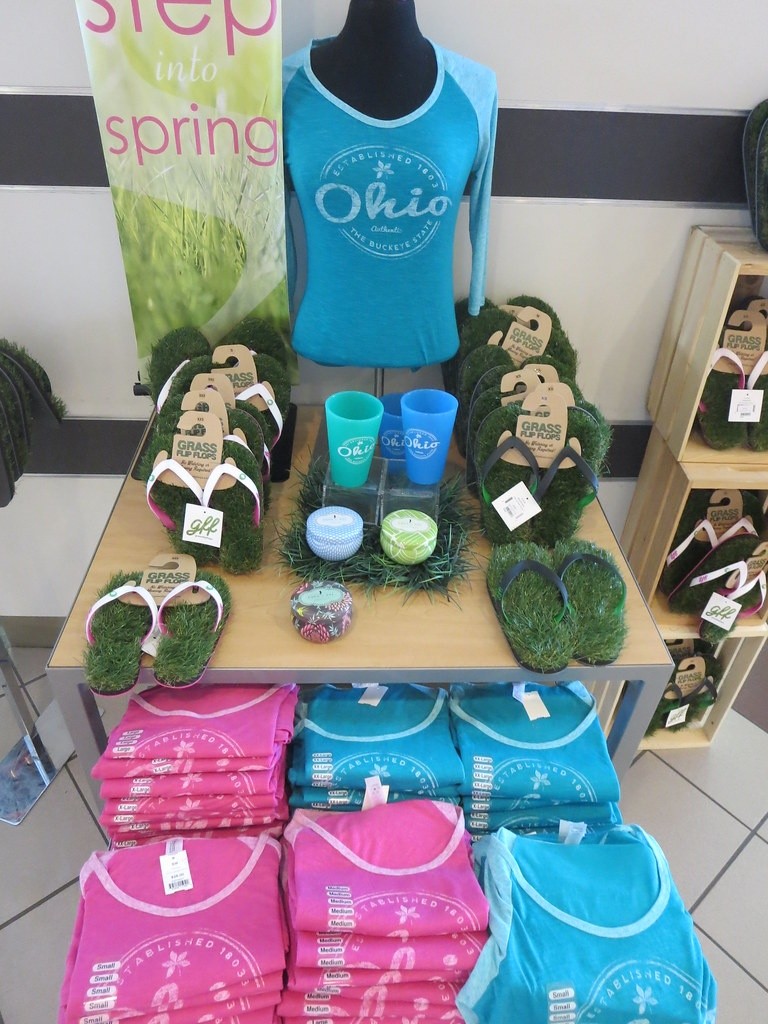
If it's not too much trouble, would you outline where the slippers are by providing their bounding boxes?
[142,315,293,574]
[646,297,768,739]
[84,572,157,696]
[441,294,626,674]
[153,571,231,688]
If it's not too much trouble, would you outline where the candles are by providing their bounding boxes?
[380,510,439,566]
[306,505,363,561]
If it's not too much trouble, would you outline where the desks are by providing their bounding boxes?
[44,402,674,819]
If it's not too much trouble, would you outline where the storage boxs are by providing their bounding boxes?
[322,455,440,525]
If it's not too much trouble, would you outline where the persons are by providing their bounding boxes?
[283,0,499,369]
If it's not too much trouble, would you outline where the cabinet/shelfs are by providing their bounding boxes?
[621,228,768,750]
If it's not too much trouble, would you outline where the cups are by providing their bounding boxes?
[400,388,459,485]
[324,390,384,488]
[378,392,406,473]
